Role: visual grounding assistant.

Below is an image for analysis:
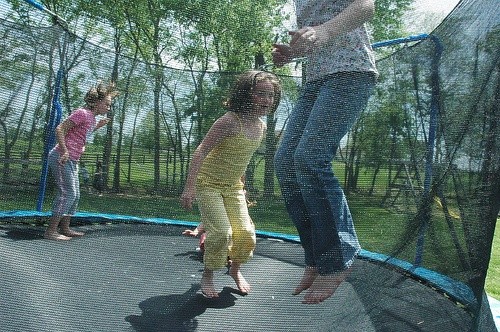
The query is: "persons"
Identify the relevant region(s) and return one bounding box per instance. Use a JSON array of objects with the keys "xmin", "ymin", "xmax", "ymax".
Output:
[
  {"xmin": 44, "ymin": 79, "xmax": 121, "ymax": 240},
  {"xmin": 183, "ymin": 215, "xmax": 236, "ymax": 265},
  {"xmin": 270, "ymin": 1, "xmax": 381, "ymax": 306},
  {"xmin": 182, "ymin": 68, "xmax": 283, "ymax": 296}
]
